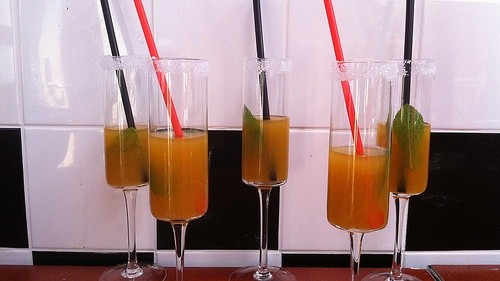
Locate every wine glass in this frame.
[228,54,297,281]
[360,57,436,281]
[323,57,392,280]
[100,53,166,281]
[146,56,209,280]
